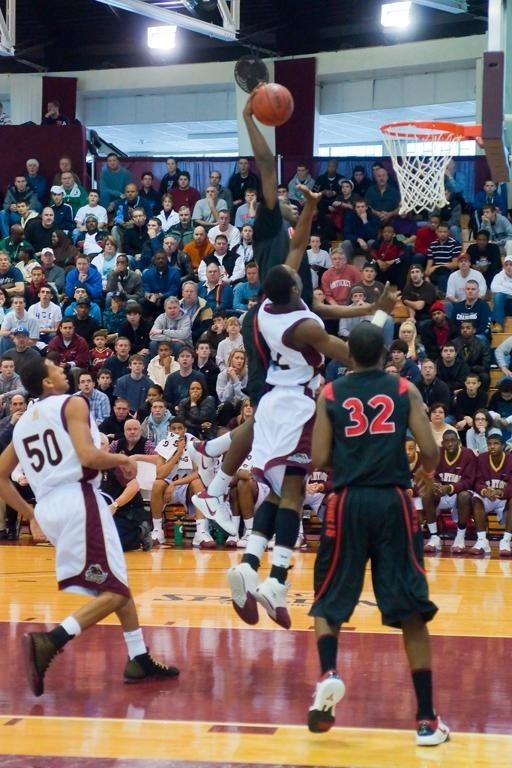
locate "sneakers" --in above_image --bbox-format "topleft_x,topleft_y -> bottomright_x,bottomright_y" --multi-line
148,529 -> 166,546
188,440 -> 219,489
124,652 -> 179,682
225,562 -> 258,624
191,487 -> 253,547
423,535 -> 512,556
308,668 -> 345,731
252,576 -> 291,628
415,715 -> 450,745
492,322 -> 503,333
24,632 -> 58,696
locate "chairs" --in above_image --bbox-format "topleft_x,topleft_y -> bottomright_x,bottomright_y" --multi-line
5,489 -> 512,546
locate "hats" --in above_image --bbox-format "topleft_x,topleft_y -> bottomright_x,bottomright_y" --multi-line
76,299 -> 90,306
504,255 -> 512,263
431,303 -> 446,313
13,327 -> 28,335
42,248 -> 53,256
458,253 -> 469,261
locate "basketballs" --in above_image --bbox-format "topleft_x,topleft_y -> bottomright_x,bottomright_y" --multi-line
250,83 -> 293,125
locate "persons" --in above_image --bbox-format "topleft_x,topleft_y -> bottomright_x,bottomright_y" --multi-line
0,357 -> 180,697
226,184 -> 351,628
308,321 -> 451,746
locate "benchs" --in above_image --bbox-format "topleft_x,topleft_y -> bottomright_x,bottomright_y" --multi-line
376,271 -> 512,413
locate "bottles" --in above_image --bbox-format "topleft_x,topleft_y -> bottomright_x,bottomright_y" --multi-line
174,519 -> 184,546
215,528 -> 225,545
